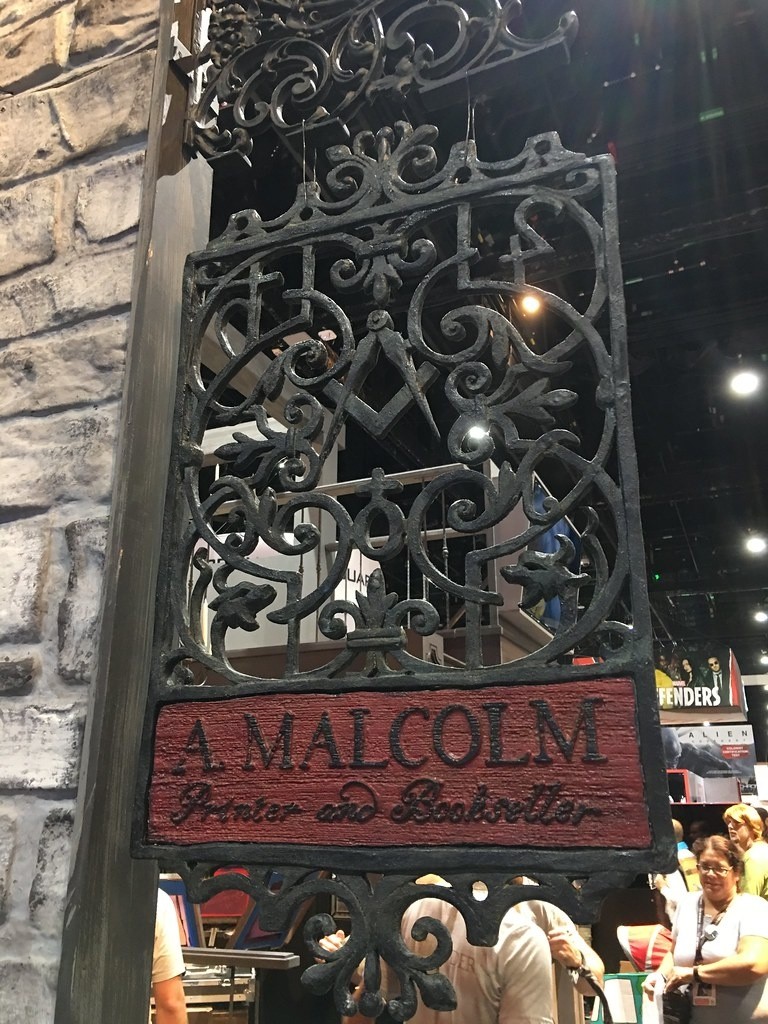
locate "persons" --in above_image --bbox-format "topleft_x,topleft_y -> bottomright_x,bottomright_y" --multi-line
146,793 -> 768,1023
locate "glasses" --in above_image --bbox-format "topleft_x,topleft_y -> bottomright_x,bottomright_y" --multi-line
695,864 -> 734,877
